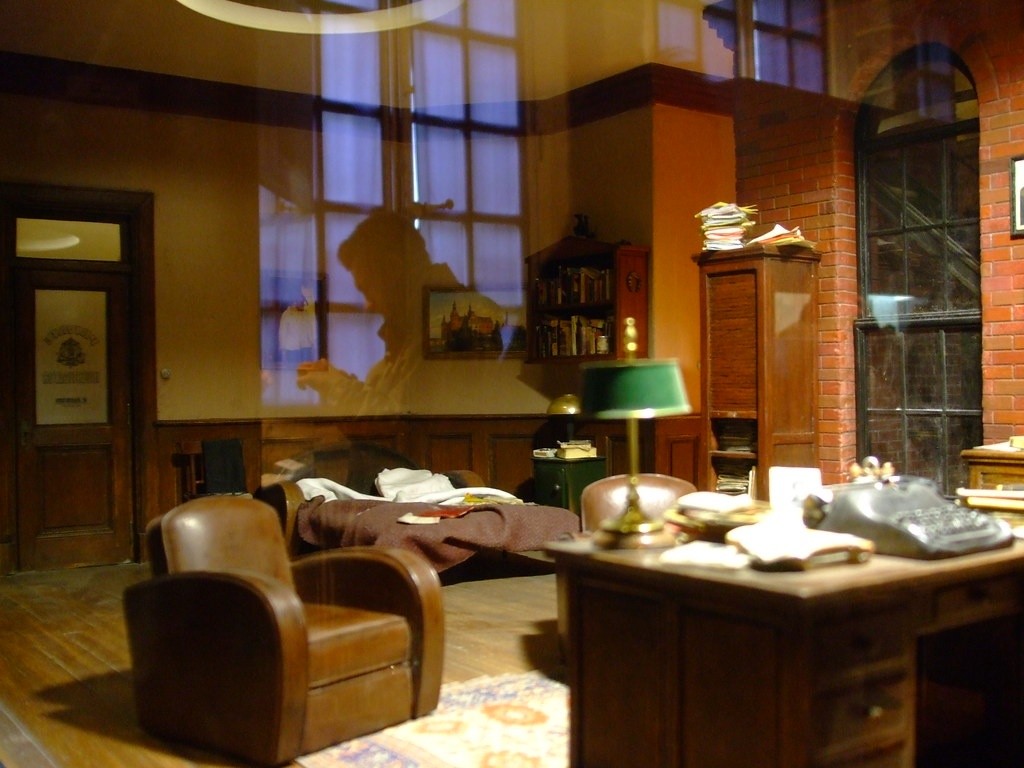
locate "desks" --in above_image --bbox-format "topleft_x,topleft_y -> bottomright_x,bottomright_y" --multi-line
960,436 -> 1024,492
543,506 -> 1024,768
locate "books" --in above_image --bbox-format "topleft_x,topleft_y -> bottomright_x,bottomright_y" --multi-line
694,201 -> 816,250
533,265 -> 615,357
715,422 -> 759,500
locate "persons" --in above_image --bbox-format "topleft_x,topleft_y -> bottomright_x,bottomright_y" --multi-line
297,211 -> 505,412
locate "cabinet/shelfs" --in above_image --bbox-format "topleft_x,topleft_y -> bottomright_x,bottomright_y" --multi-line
686,244 -> 824,500
520,234 -> 649,367
531,455 -> 607,519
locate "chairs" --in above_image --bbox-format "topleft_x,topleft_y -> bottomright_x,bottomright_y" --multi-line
580,473 -> 697,533
176,436 -> 249,505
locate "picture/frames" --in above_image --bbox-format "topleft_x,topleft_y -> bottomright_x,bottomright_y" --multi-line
423,283 -> 528,360
259,266 -> 329,371
1008,155 -> 1024,239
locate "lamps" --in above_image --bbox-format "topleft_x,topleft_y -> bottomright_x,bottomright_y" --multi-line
545,393 -> 585,443
579,318 -> 694,558
277,217 -> 322,353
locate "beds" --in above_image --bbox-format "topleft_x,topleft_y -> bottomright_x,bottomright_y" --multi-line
255,438 -> 579,574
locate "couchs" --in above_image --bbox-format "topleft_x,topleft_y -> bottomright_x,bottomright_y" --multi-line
119,494 -> 444,764
